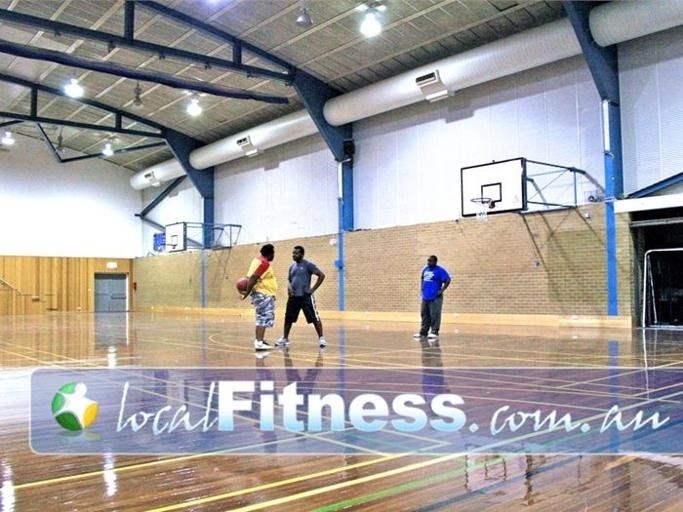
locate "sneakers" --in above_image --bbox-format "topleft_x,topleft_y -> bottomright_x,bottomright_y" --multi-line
319,336 -> 327,347
413,333 -> 438,338
254,337 -> 290,351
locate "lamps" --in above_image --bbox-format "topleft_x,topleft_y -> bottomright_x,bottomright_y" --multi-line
55,135 -> 65,152
132,83 -> 143,107
295,8 -> 314,27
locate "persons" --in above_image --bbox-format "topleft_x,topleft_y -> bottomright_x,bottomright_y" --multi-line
249,350 -> 279,456
280,346 -> 326,443
412,336 -> 451,431
412,255 -> 451,338
237,244 -> 276,350
273,245 -> 328,348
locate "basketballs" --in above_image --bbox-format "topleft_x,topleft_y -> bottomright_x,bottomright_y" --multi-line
236,278 -> 250,291
